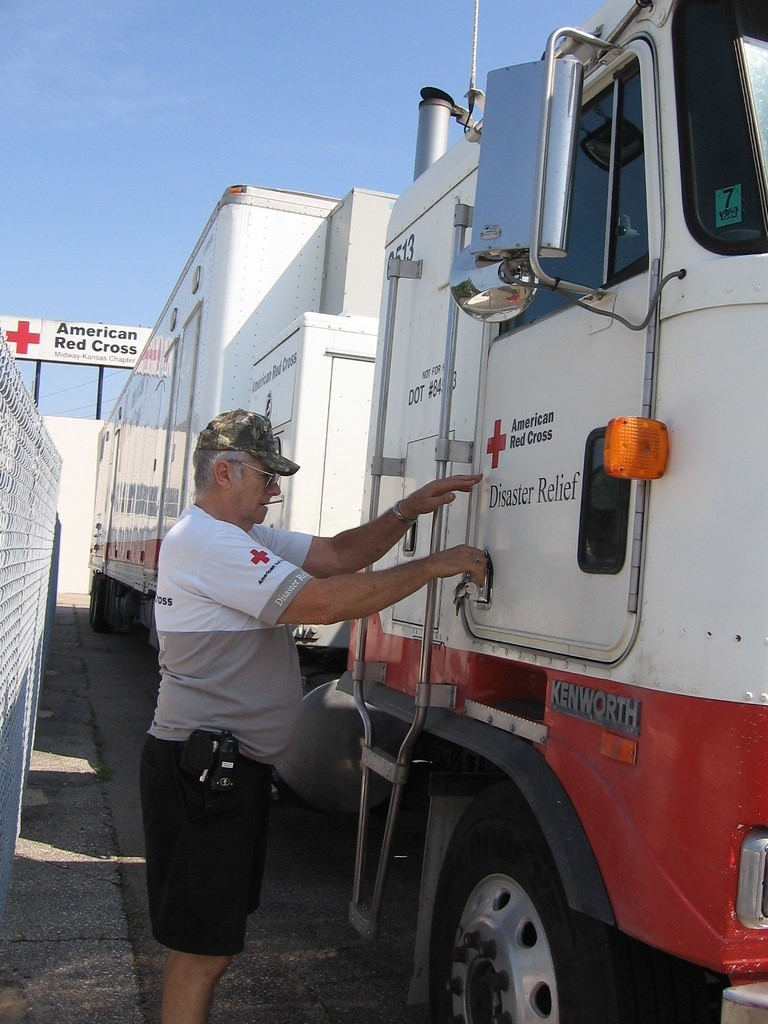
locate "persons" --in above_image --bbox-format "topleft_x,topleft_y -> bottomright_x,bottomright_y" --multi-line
140,408 -> 490,1024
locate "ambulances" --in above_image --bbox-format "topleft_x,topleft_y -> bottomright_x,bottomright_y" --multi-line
84,1 -> 768,1023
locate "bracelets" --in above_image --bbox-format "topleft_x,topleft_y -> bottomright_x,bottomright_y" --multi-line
393,498 -> 418,525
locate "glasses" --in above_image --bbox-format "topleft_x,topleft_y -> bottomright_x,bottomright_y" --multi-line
228,459 -> 280,488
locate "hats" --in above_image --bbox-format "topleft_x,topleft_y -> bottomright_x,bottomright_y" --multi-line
196,408 -> 300,476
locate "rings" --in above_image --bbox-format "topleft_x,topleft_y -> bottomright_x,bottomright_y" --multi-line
475,559 -> 480,565
